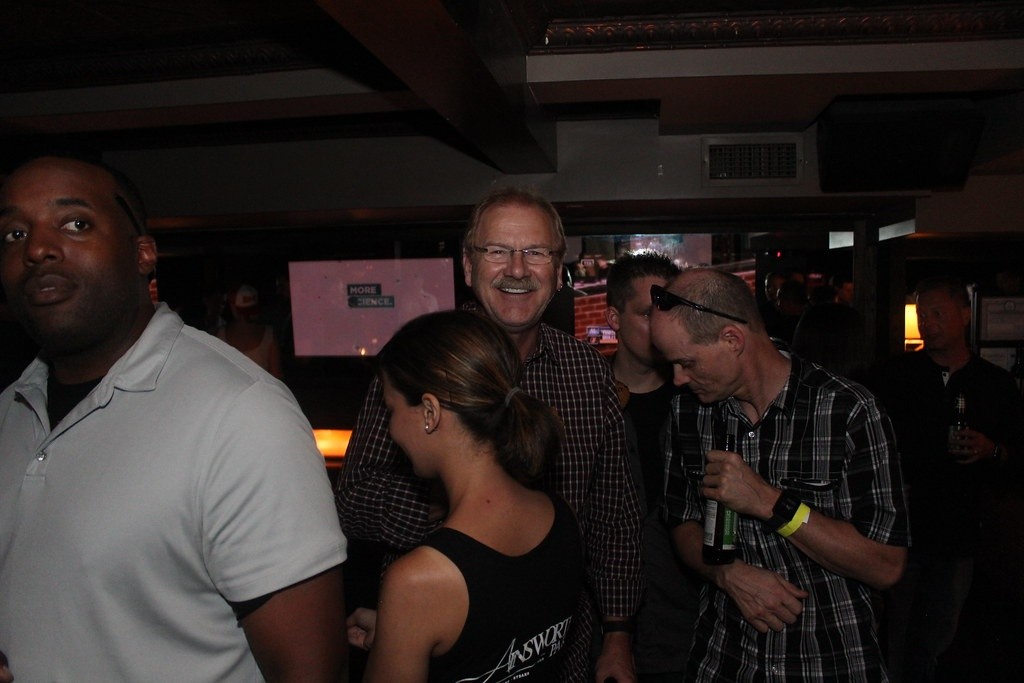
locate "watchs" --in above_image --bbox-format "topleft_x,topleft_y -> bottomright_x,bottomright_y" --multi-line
759,491 -> 800,536
988,441 -> 1002,464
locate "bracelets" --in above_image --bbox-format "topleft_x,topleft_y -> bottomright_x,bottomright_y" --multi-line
601,622 -> 636,636
776,502 -> 809,536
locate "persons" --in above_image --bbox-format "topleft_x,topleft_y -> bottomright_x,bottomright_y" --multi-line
216,282 -> 284,378
0,154 -> 348,683
347,311 -> 582,683
650,270 -> 912,682
762,271 -> 1024,683
605,254 -> 701,683
338,187 -> 646,683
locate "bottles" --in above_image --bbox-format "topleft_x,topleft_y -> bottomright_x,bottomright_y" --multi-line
702,434 -> 738,566
949,394 -> 968,461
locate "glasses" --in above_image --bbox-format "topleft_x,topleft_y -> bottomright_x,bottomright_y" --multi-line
474,245 -> 562,265
649,284 -> 748,324
